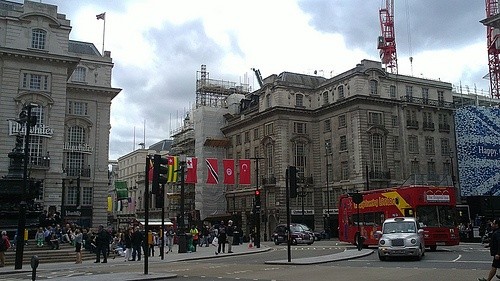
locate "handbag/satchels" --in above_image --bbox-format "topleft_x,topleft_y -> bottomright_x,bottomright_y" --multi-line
193,234 -> 198,240
212,237 -> 218,246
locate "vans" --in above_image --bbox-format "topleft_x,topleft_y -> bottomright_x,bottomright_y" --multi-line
375,217 -> 425,261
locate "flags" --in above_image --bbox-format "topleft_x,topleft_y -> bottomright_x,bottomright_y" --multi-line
205,158 -> 219,184
185,157 -> 198,183
223,159 -> 235,185
96,13 -> 104,20
239,159 -> 250,185
166,156 -> 179,182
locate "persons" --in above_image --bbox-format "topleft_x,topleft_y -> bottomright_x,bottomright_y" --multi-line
458,220 -> 495,249
477,219 -> 500,281
148,220 -> 254,257
0,229 -> 18,267
24,210 -> 146,264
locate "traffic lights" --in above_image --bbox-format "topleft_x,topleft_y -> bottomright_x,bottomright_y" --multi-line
151,153 -> 169,194
254,190 -> 260,205
289,165 -> 301,198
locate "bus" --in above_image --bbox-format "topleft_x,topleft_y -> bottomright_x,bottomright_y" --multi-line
338,184 -> 463,251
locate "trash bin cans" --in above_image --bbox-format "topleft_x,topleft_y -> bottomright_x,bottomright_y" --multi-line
179,233 -> 196,253
232,232 -> 239,245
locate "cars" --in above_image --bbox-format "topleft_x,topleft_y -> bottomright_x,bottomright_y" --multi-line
271,223 -> 315,246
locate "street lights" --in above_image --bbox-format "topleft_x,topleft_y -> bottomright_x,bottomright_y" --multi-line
13,102 -> 39,270
178,150 -> 187,253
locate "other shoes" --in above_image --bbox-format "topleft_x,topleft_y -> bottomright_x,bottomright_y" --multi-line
101,261 -> 107,263
228,251 -> 233,253
41,244 -> 43,247
37,245 -> 40,247
94,261 -> 100,263
130,259 -> 135,261
75,260 -> 82,264
137,259 -> 141,261
